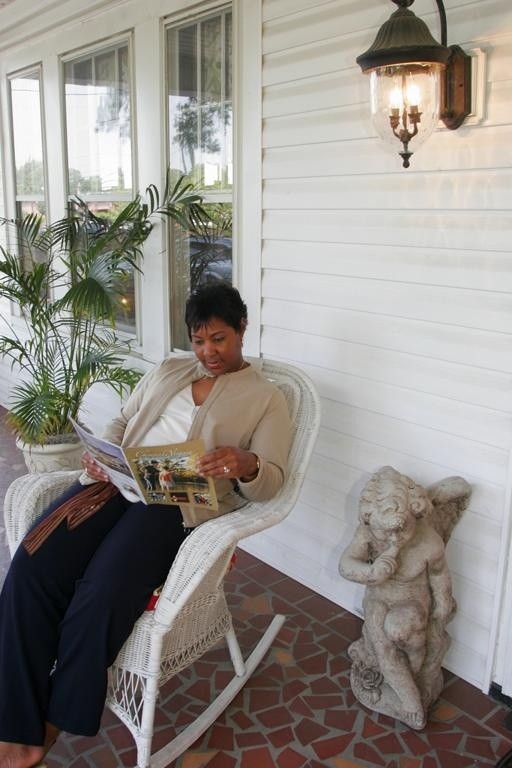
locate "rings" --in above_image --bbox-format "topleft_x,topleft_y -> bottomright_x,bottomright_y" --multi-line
223,465 -> 230,473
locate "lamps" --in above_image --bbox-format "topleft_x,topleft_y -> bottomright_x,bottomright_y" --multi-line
357,0 -> 473,168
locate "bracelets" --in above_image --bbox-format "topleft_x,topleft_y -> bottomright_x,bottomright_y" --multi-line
243,453 -> 260,480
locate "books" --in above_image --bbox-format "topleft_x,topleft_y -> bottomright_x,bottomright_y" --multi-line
69,415 -> 219,513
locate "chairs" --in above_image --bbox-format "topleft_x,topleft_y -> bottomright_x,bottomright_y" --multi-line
2,357 -> 324,767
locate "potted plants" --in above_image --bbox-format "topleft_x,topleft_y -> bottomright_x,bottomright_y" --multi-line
0,162 -> 216,477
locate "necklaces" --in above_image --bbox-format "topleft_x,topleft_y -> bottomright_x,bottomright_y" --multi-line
238,360 -> 246,370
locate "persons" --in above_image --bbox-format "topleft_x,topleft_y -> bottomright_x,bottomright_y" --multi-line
138,460 -> 160,491
338,465 -> 472,728
0,285 -> 295,767
159,465 -> 177,491
